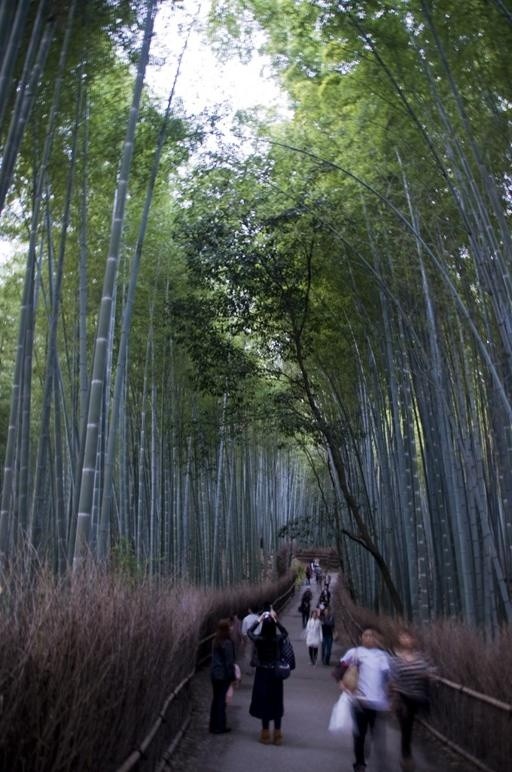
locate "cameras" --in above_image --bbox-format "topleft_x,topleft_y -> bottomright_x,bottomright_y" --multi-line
263,611 -> 270,619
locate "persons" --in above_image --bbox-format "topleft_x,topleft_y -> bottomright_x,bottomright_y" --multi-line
305,555 -> 331,588
209,618 -> 237,734
243,604 -> 264,674
320,606 -> 336,666
319,583 -> 331,607
231,611 -> 242,661
390,627 -> 431,771
247,608 -> 289,744
300,585 -> 312,628
305,610 -> 323,669
337,624 -> 396,771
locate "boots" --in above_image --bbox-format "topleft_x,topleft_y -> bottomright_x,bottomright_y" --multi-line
257,729 -> 272,746
272,729 -> 284,746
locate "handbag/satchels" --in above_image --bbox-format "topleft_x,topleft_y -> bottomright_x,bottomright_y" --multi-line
342,662 -> 360,692
270,660 -> 291,681
327,690 -> 356,737
282,635 -> 296,670
